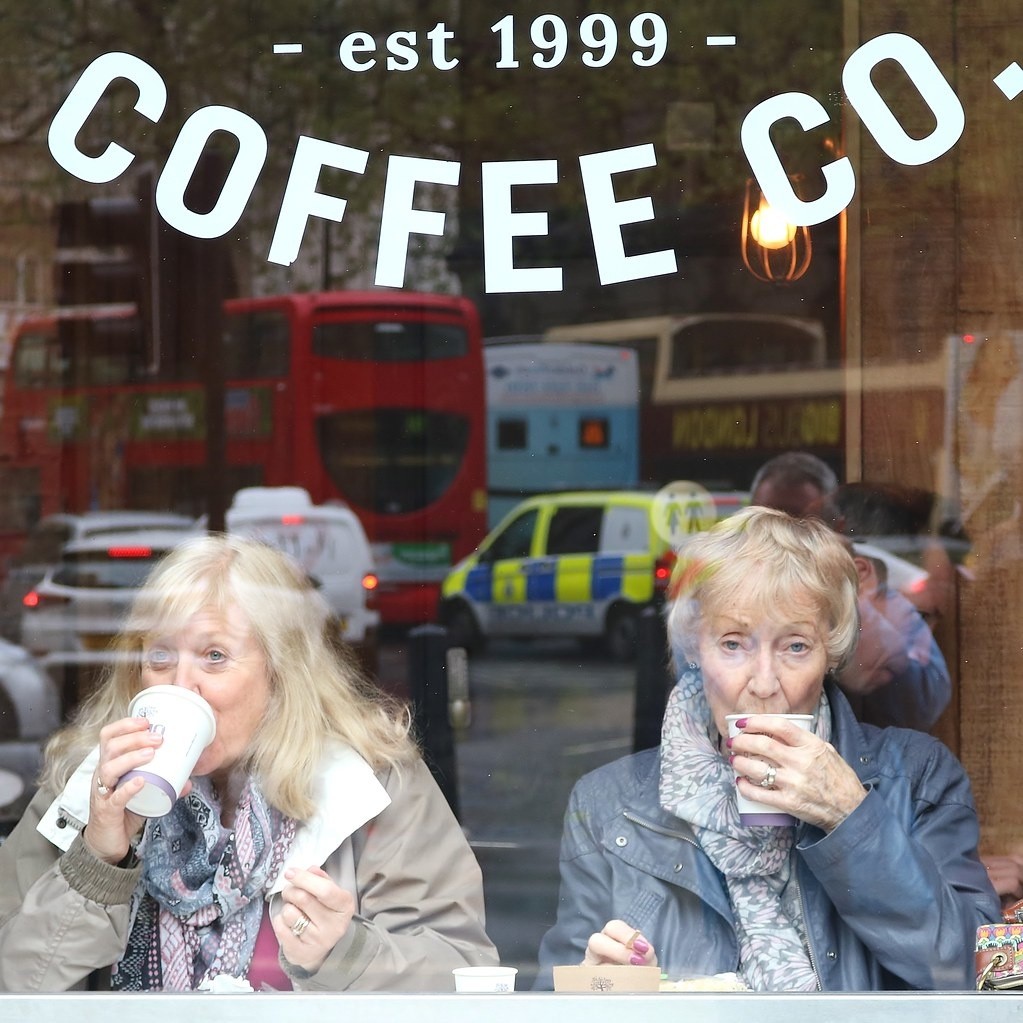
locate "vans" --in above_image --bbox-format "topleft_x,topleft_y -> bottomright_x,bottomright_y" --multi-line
437,491 -> 753,661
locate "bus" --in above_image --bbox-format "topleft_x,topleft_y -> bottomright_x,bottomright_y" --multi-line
0,291 -> 485,640
484,344 -> 642,537
546,313 -> 831,492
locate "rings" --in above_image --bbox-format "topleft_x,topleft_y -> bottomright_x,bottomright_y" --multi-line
761,765 -> 776,786
292,914 -> 311,935
97,777 -> 107,795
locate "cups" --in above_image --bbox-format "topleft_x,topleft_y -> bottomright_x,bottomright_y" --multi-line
724,714 -> 815,827
451,967 -> 517,993
114,685 -> 217,817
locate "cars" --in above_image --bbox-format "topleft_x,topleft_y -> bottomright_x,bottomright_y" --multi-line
0,510 -> 207,668
225,485 -> 379,674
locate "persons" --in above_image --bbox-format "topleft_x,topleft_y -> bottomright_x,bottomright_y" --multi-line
0,537 -> 500,995
750,454 -> 974,729
529,505 -> 1003,991
